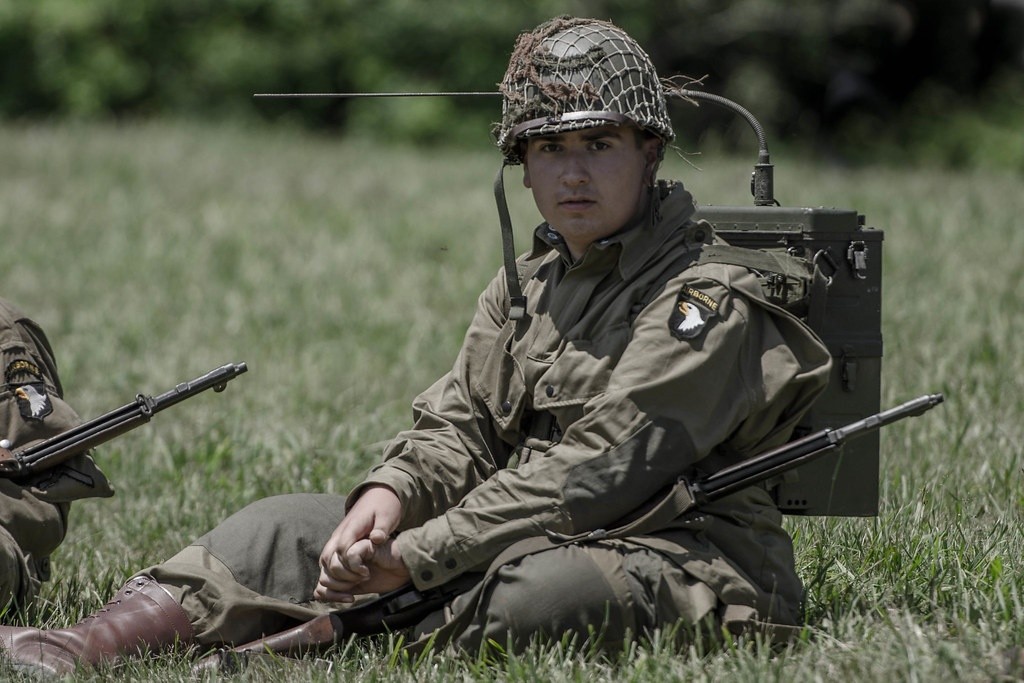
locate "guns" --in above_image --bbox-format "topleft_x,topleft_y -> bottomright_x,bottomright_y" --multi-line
0,362 -> 251,490
187,392 -> 948,676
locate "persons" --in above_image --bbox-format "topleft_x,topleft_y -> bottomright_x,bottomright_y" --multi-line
1,14 -> 833,681
0,299 -> 113,629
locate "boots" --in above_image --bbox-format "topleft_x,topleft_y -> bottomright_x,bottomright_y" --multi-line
0,577 -> 191,681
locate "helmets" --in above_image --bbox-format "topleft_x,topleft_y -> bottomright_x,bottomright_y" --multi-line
495,19 -> 674,161
